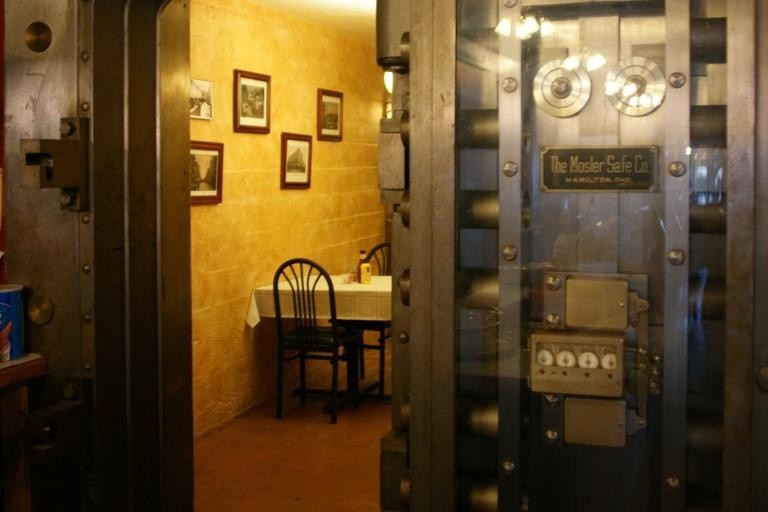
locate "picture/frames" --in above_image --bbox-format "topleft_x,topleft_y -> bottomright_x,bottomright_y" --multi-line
317,88 -> 343,142
189,79 -> 214,119
281,133 -> 312,189
191,142 -> 223,205
234,69 -> 270,135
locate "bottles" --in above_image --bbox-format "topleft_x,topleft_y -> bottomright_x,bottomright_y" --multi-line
352,249 -> 372,285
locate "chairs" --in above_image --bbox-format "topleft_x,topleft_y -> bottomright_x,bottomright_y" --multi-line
361,243 -> 391,381
273,258 -> 356,424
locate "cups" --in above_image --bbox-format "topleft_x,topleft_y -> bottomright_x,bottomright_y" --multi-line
0,284 -> 24,363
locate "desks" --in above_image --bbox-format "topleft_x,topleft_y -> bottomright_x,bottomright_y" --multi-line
245,273 -> 392,414
1,353 -> 48,512
